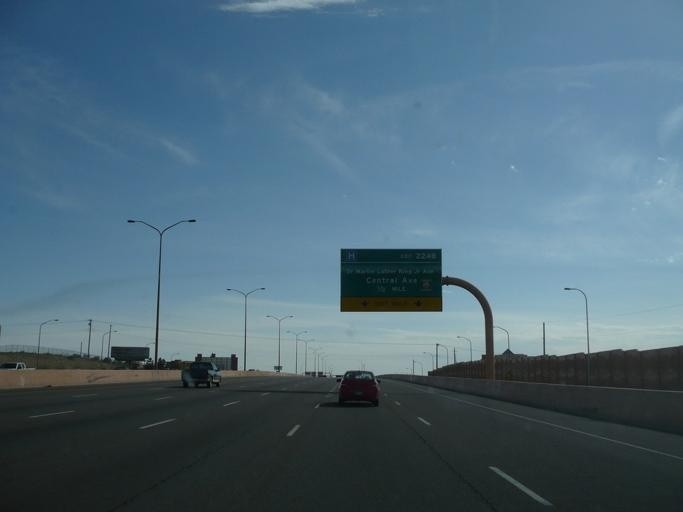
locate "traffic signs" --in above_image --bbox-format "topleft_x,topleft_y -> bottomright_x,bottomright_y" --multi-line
339,247 -> 443,313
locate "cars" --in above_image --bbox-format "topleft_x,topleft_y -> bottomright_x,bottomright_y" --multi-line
335,369 -> 383,408
248,368 -> 256,371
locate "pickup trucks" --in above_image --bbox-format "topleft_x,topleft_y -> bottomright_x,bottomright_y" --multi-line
0,361 -> 36,370
178,360 -> 222,390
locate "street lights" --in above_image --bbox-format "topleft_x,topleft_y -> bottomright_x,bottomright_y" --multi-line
226,287 -> 266,371
170,352 -> 180,361
563,287 -> 589,355
36,317 -> 120,364
266,314 -> 295,372
283,328 -> 335,378
144,342 -> 156,365
492,326 -> 510,350
455,336 -> 473,362
123,216 -> 198,369
404,342 -> 449,377
